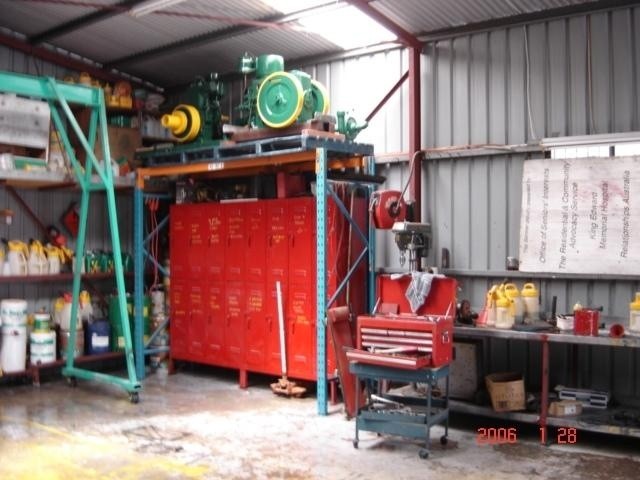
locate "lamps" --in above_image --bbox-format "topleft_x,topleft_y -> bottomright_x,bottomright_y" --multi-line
127,1 -> 184,18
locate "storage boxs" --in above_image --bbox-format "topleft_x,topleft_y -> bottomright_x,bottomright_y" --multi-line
485,371 -> 525,413
76,124 -> 142,175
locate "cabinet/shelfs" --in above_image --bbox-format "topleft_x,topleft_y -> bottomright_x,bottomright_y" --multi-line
379,321 -> 639,444
133,134 -> 384,415
352,362 -> 450,459
169,197 -> 367,404
2,167 -> 170,387
